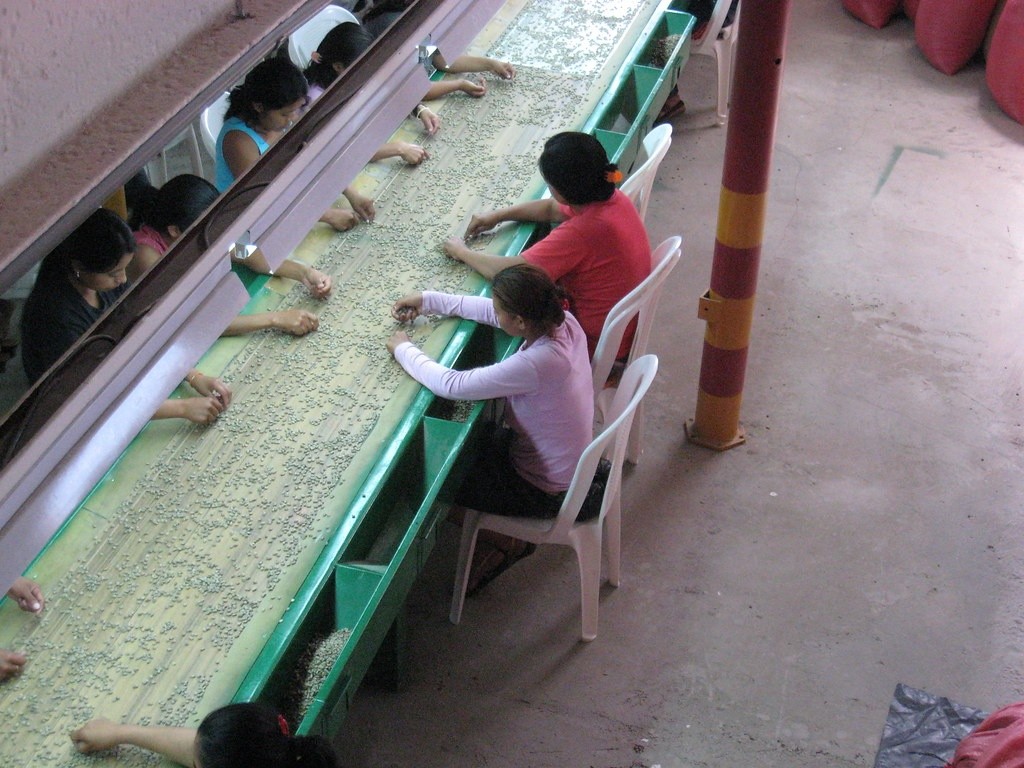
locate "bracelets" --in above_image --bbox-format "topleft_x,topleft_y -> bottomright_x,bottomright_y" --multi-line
413,103 -> 430,120
189,372 -> 203,388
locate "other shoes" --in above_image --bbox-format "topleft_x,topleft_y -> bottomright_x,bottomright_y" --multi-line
448,540 -> 537,598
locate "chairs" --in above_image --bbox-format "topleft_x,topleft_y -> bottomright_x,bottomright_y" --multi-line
199,92 -> 231,163
449,354 -> 658,642
144,124 -> 205,189
288,4 -> 360,70
591,236 -> 683,464
691,0 -> 742,126
618,123 -> 673,221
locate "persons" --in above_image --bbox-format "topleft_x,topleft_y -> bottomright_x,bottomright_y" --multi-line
301,21 -> 440,165
213,57 -> 375,232
70,702 -> 337,768
20,208 -> 232,427
0,576 -> 45,682
362,0 -> 517,101
443,131 -> 652,376
386,263 -> 595,521
127,174 -> 332,336
